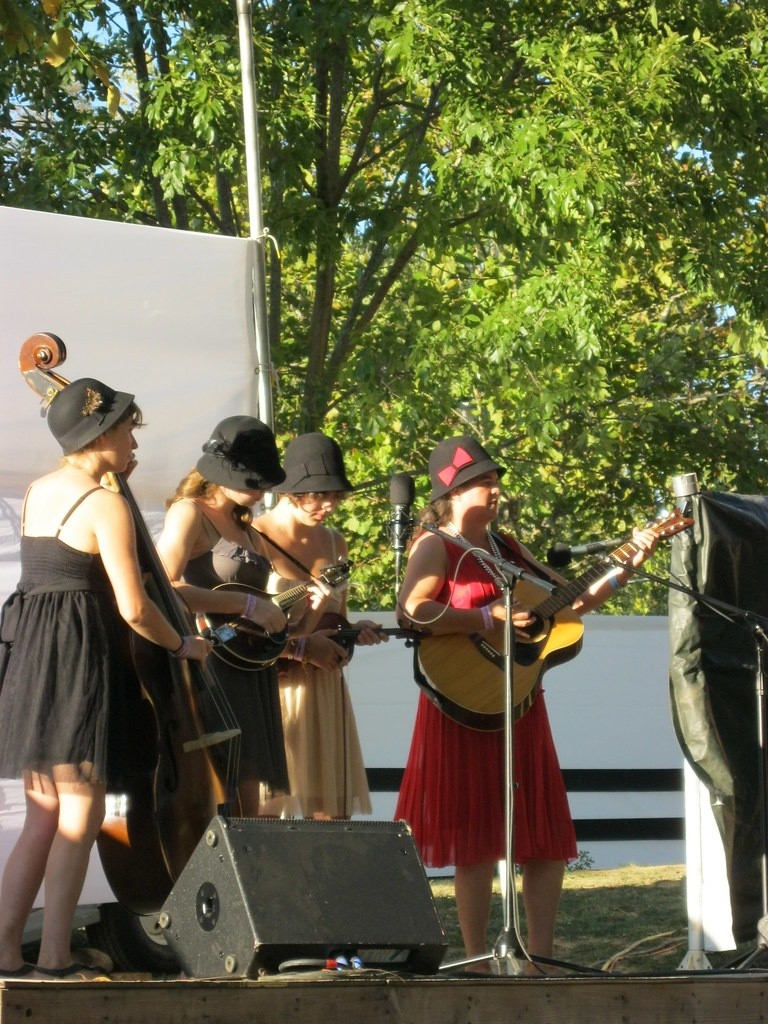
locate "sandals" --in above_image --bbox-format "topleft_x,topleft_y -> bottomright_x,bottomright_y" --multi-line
34,962 -> 110,979
0,962 -> 34,979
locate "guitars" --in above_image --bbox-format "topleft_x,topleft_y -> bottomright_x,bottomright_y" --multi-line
193,555 -> 359,673
411,511 -> 695,734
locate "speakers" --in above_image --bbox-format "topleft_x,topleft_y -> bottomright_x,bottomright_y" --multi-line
155,816 -> 451,979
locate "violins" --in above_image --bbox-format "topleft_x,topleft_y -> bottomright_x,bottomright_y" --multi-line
264,611 -> 429,674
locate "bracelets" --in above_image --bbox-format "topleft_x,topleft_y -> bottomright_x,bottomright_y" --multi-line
478,604 -> 496,634
287,639 -> 296,660
606,569 -> 624,591
242,594 -> 256,619
169,636 -> 191,659
294,637 -> 305,661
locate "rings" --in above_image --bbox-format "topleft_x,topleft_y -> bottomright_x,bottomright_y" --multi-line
205,653 -> 208,656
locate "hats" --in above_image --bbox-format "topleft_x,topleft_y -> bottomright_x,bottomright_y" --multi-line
47,377 -> 135,456
270,433 -> 354,493
196,415 -> 287,490
428,436 -> 507,503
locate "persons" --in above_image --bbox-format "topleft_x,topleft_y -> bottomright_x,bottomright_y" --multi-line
393,436 -> 659,976
153,415 -> 332,819
0,378 -> 212,983
253,432 -> 388,970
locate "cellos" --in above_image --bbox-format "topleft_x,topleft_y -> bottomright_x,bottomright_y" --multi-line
17,330 -> 262,918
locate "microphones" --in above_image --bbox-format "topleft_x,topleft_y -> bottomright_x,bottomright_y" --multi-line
390,473 -> 415,575
545,537 -> 624,567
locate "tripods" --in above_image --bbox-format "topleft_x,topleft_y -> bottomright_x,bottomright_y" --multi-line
590,551 -> 768,974
408,516 -> 608,974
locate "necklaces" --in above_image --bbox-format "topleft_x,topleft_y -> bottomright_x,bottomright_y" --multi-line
445,522 -> 505,576
